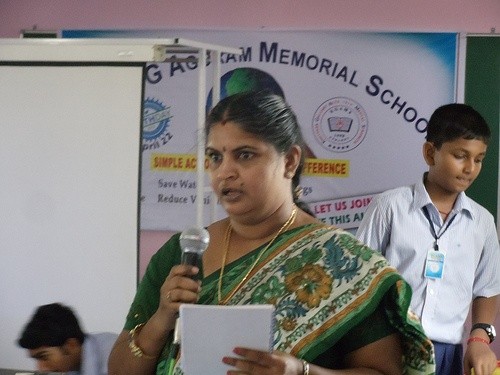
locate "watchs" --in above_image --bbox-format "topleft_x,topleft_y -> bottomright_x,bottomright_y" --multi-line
470,323 -> 496,344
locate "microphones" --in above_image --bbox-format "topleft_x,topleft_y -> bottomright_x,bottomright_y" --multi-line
171,225 -> 211,360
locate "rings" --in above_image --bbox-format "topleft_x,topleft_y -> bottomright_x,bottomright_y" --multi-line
167,290 -> 171,301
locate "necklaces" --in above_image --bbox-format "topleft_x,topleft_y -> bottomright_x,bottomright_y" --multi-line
439,210 -> 449,216
217,203 -> 297,305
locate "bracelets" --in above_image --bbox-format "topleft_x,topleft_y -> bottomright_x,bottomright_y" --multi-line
127,323 -> 159,360
467,337 -> 489,345
301,359 -> 310,375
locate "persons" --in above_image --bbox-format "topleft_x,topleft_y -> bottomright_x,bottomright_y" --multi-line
357,103 -> 500,375
108,89 -> 437,375
17,303 -> 120,375
